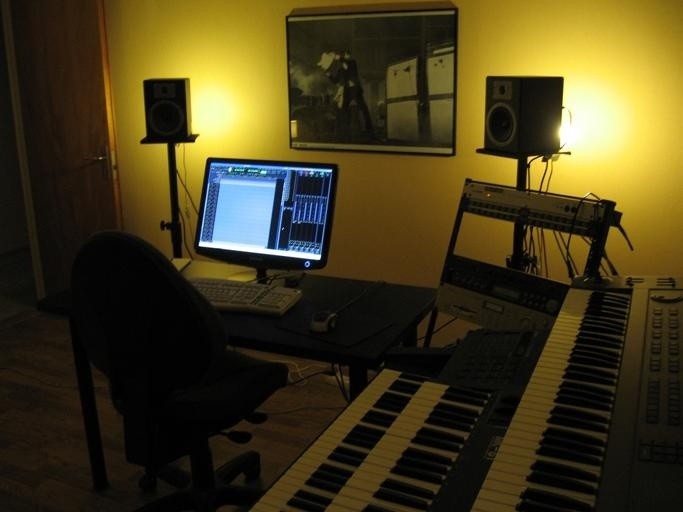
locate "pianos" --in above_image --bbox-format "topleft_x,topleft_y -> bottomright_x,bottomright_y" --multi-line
249,285 -> 682,511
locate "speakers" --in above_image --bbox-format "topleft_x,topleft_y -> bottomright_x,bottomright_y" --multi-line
144,78 -> 192,139
485,76 -> 564,156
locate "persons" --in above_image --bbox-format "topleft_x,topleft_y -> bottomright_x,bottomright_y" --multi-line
324,48 -> 376,134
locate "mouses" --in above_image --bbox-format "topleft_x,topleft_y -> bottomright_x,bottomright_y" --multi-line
310,310 -> 338,333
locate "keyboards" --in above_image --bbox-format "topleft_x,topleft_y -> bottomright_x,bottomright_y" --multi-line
185,278 -> 303,316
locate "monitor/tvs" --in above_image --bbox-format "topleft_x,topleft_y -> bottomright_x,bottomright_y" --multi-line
193,157 -> 338,285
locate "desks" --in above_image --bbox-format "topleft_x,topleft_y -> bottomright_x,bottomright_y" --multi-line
66,255 -> 442,494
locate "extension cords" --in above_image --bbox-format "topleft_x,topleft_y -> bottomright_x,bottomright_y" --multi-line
325,374 -> 349,390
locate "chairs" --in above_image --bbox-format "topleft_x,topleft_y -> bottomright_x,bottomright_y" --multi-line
66,226 -> 288,512
